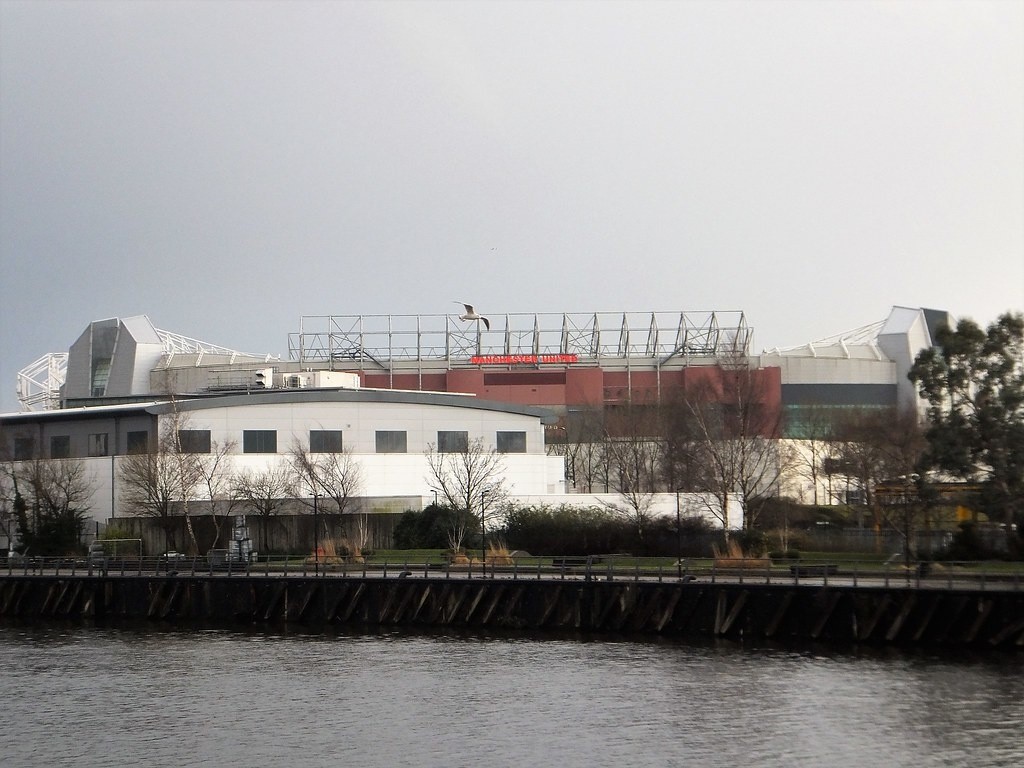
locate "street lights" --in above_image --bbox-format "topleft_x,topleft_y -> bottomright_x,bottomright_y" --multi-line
164,498 -> 173,575
430,489 -> 437,507
676,487 -> 684,581
309,492 -> 322,576
482,490 -> 490,575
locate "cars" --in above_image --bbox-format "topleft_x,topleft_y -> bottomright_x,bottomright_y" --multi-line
164,551 -> 180,558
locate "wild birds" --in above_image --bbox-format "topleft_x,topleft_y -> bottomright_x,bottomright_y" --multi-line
453,301 -> 490,332
